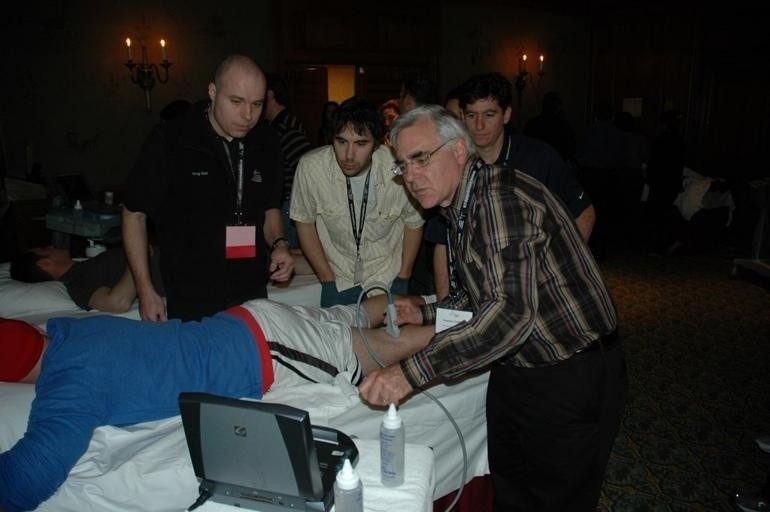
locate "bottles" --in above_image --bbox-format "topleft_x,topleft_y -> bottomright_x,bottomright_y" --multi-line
333,456 -> 364,512
72,200 -> 84,224
380,403 -> 407,488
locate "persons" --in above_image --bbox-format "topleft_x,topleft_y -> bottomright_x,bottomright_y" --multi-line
260,70 -> 315,251
1,285 -> 446,511
118,51 -> 296,323
9,242 -> 171,312
290,71 -> 656,321
353,103 -> 621,509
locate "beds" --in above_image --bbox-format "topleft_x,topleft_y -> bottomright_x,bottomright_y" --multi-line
12,256 -> 325,321
1,307 -> 494,511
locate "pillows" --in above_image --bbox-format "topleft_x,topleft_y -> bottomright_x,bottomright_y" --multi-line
0,261 -> 81,316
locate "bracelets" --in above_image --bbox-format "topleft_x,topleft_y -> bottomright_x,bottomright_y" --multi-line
271,235 -> 287,248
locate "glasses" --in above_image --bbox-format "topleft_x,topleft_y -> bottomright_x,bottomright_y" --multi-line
390,142 -> 449,175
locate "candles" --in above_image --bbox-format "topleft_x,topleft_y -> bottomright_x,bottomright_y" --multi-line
539,55 -> 544,71
522,54 -> 527,72
126,37 -> 132,60
160,38 -> 166,60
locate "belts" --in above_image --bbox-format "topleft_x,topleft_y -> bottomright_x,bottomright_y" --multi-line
574,343 -> 599,356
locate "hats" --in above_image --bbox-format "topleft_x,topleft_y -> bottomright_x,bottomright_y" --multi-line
1,318 -> 44,383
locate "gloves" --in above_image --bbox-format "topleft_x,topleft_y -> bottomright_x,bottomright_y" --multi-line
320,281 -> 344,309
390,275 -> 409,296
